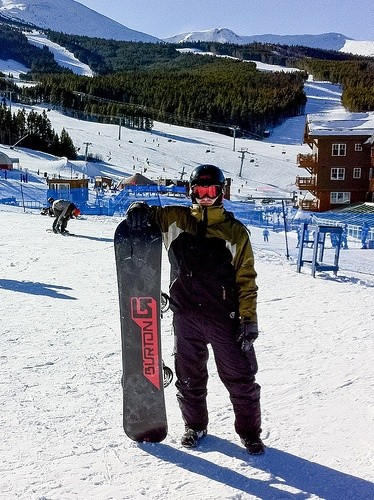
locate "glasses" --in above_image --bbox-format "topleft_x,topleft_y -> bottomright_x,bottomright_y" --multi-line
192,185 -> 222,199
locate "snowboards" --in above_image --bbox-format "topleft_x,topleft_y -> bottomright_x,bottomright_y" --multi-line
109,213 -> 170,444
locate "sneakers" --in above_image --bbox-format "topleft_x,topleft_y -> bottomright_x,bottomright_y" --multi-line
241,434 -> 265,455
181,425 -> 208,448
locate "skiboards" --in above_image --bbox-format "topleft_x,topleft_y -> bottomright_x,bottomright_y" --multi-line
46,228 -> 73,237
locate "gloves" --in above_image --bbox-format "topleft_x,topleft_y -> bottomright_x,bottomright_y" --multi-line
125,199 -> 152,232
236,322 -> 259,351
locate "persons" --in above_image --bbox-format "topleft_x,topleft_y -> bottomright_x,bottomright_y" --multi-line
53,200 -> 79,236
263,227 -> 269,242
296,223 -> 348,249
126,165 -> 265,455
358,222 -> 370,249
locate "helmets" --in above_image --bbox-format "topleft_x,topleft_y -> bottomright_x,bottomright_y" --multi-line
75,209 -> 80,215
185,163 -> 229,192
